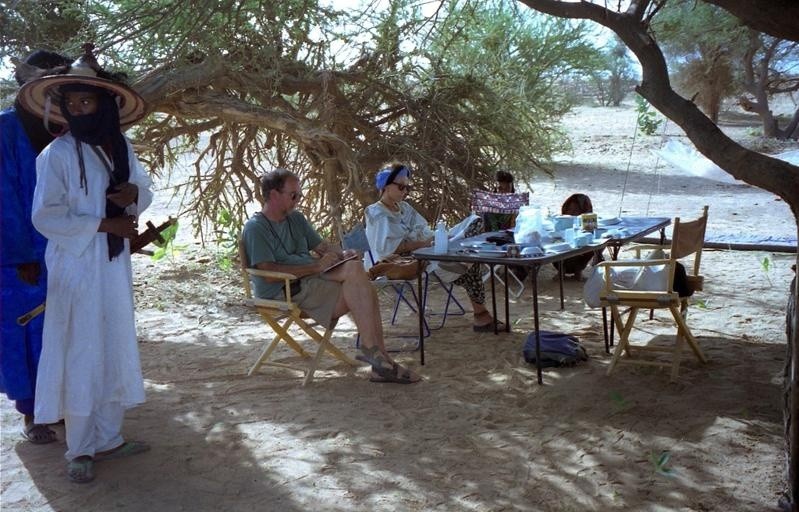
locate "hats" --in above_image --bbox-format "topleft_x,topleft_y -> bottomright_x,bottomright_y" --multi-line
17,42 -> 147,129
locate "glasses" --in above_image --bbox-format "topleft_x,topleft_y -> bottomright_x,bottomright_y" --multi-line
277,188 -> 303,201
392,181 -> 412,191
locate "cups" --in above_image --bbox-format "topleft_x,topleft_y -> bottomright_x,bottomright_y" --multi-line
564,228 -> 575,242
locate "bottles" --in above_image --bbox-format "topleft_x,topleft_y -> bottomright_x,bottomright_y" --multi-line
434,219 -> 448,255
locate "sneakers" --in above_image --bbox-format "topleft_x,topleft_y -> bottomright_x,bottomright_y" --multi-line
438,261 -> 467,274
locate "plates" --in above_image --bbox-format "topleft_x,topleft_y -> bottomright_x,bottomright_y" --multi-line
477,250 -> 508,257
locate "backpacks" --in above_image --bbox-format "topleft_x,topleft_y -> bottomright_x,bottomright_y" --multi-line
523,327 -> 588,370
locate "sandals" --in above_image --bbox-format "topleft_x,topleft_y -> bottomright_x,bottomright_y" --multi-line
355,344 -> 396,372
369,365 -> 421,384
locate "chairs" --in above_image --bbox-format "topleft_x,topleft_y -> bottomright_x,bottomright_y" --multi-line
344,223 -> 463,337
235,230 -> 356,388
469,186 -> 530,300
583,204 -> 713,383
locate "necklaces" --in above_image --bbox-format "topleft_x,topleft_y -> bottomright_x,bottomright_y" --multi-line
259,209 -> 302,258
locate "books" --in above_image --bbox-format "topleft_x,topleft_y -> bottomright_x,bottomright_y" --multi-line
323,255 -> 357,273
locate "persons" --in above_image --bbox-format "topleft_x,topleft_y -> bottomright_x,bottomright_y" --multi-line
360,162 -> 509,333
477,170 -> 531,288
552,193 -> 603,281
238,168 -> 423,386
0,102 -> 70,448
31,82 -> 156,486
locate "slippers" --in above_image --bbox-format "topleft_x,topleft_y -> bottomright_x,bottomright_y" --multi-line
19,420 -> 57,444
102,440 -> 152,460
473,319 -> 510,332
65,460 -> 95,483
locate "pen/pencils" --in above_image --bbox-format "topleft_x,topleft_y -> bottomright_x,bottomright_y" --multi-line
313,249 -> 324,257
